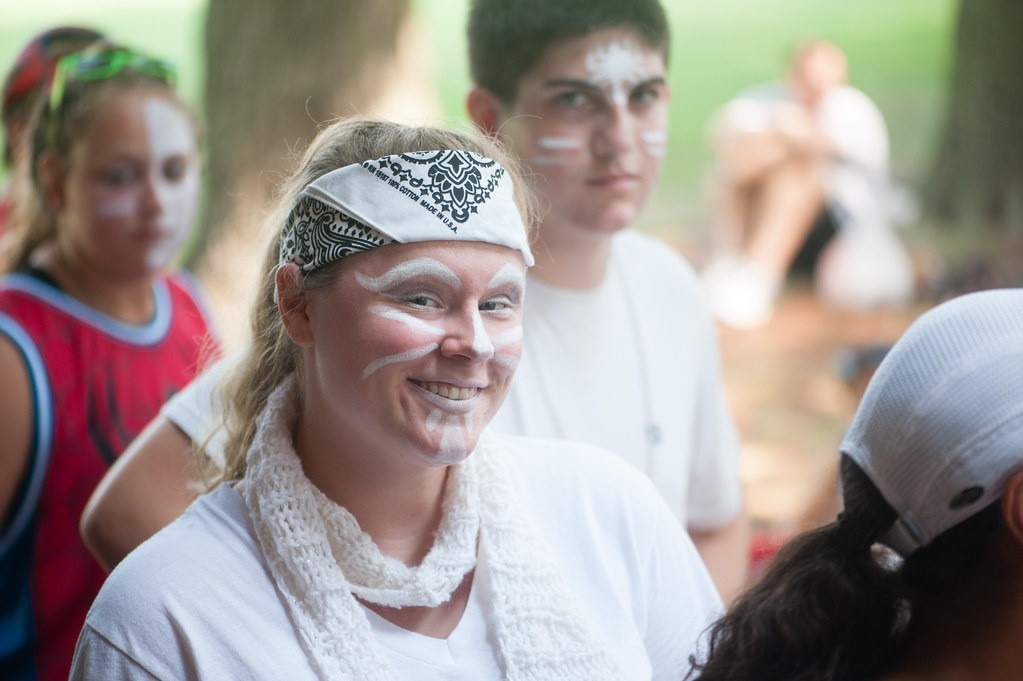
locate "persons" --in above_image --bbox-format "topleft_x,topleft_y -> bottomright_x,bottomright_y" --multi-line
66,121 -> 728,681
705,42 -> 890,325
89,0 -> 749,610
695,290 -> 1023,681
0,27 -> 223,681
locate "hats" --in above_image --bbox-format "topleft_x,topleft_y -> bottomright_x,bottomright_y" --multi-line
838,287 -> 1022,571
